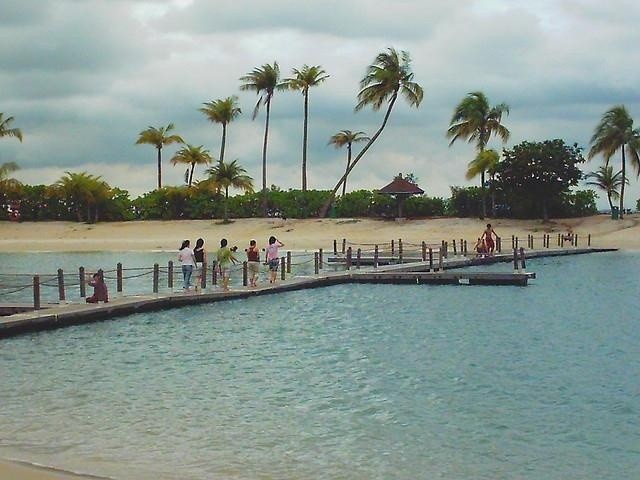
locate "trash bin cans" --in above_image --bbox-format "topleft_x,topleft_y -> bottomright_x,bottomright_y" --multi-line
330,204 -> 338,218
612,206 -> 619,219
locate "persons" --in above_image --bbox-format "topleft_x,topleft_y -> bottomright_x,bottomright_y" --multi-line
265,236 -> 285,283
177,240 -> 198,288
480,224 -> 498,255
193,238 -> 208,284
474,237 -> 487,253
86,273 -> 108,303
246,240 -> 260,287
216,238 -> 234,291
